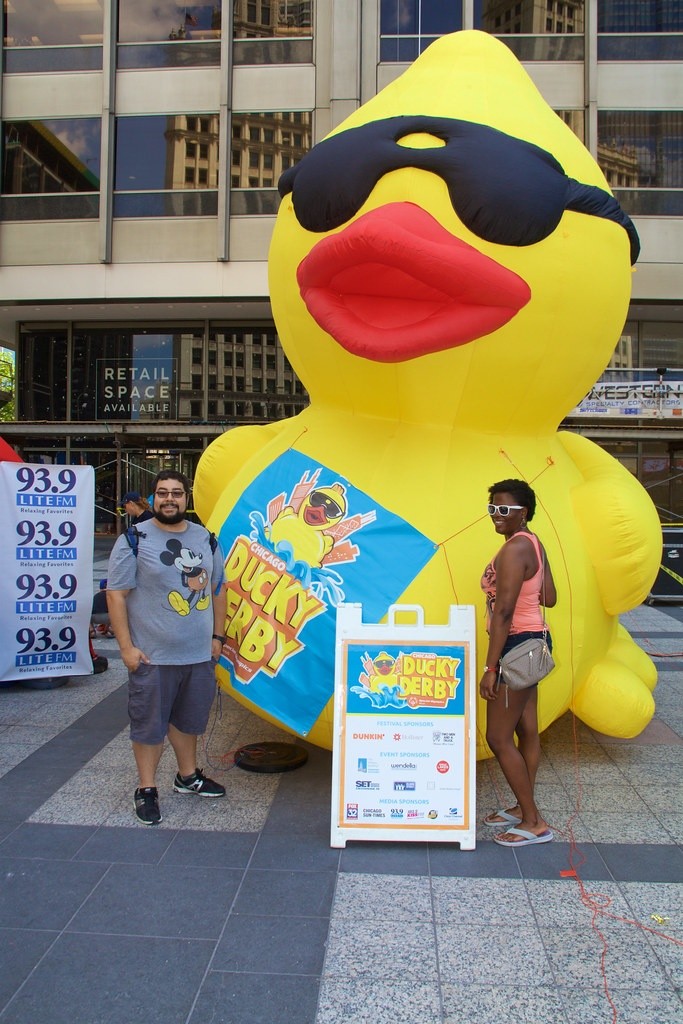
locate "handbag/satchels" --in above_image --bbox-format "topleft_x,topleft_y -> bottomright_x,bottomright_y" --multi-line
498,639 -> 555,691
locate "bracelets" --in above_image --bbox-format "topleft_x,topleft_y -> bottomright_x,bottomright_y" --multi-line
213,634 -> 225,645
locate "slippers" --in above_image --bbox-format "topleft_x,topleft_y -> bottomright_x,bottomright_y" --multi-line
483,808 -> 523,826
494,826 -> 554,846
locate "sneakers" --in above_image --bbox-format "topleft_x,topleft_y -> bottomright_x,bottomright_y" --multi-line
133,785 -> 163,825
171,767 -> 225,797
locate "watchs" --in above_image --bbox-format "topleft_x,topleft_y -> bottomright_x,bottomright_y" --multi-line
484,666 -> 498,673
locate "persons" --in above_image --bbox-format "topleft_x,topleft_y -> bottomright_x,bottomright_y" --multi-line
106,471 -> 227,825
115,493 -> 155,522
479,479 -> 558,848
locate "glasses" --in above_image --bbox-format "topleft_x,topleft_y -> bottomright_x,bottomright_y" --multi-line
153,489 -> 186,498
487,503 -> 522,517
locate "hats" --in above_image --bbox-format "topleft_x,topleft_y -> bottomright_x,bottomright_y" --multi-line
115,491 -> 141,505
